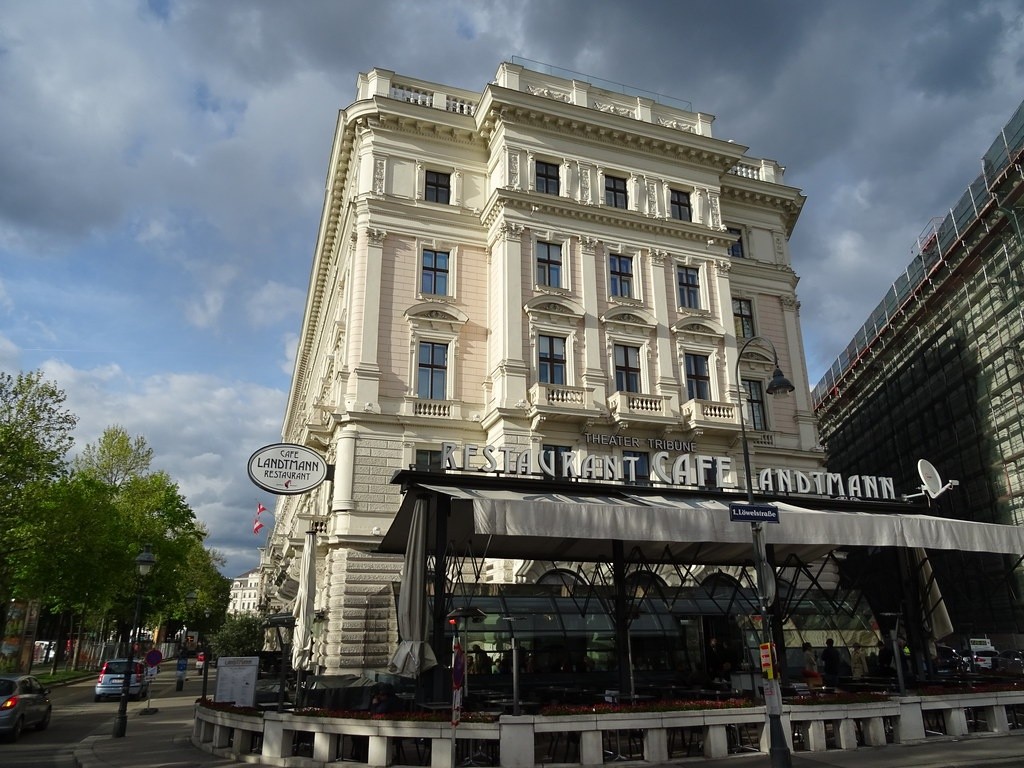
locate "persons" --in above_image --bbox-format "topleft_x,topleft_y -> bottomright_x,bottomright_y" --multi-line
877,640 -> 892,666
196,652 -> 204,676
802,641 -> 818,672
891,639 -> 911,672
819,638 -> 840,674
465,645 -> 527,674
851,642 -> 863,677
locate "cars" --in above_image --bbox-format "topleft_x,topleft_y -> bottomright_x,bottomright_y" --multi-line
973,650 -> 1000,672
990,649 -> 1024,677
0,673 -> 52,743
938,645 -> 972,673
937,660 -> 963,680
94,659 -> 148,701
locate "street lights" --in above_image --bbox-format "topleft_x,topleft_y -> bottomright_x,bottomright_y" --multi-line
202,607 -> 212,650
735,334 -> 797,768
175,589 -> 199,690
111,550 -> 157,736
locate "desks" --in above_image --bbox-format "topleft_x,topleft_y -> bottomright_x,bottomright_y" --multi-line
252,673 -> 1024,767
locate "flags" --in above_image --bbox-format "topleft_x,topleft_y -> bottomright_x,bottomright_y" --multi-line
253,518 -> 264,535
257,504 -> 265,515
